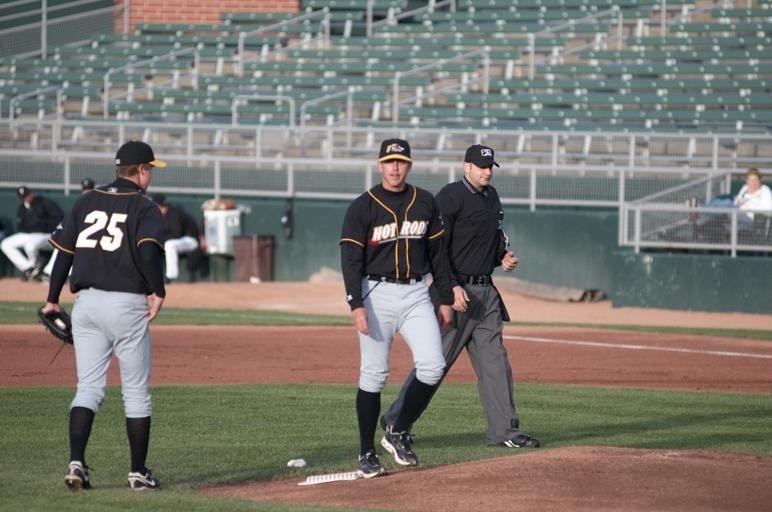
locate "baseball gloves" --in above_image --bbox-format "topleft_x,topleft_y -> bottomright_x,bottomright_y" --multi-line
39,303 -> 74,345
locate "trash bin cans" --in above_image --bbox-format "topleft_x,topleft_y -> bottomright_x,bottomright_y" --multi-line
233,235 -> 273,281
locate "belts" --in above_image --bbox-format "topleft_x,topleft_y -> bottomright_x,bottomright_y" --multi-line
368,274 -> 422,284
457,274 -> 490,287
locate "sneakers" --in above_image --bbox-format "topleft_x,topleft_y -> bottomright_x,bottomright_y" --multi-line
380,426 -> 418,467
64,461 -> 94,490
502,435 -> 540,448
380,415 -> 416,444
127,469 -> 159,491
357,450 -> 386,479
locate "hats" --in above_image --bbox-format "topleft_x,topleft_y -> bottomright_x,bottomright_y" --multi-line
378,138 -> 413,163
82,178 -> 94,190
16,186 -> 28,199
114,141 -> 166,168
464,144 -> 500,167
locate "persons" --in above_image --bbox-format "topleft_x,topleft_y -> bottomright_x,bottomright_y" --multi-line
145,193 -> 200,286
39,137 -> 168,492
338,136 -> 454,480
683,166 -> 770,234
0,185 -> 65,284
40,178 -> 96,282
378,142 -> 540,449
